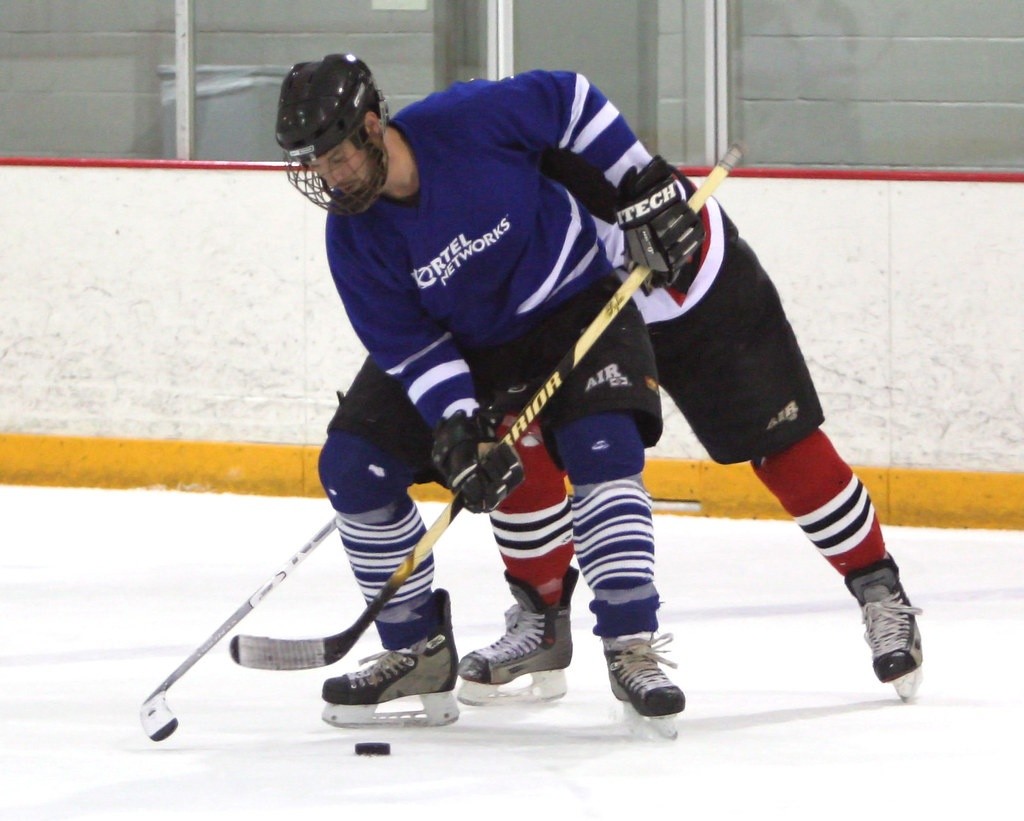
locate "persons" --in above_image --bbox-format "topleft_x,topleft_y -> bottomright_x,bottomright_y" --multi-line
274,50 -> 709,717
457,166 -> 924,687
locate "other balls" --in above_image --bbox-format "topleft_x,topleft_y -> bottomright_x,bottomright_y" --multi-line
354,741 -> 391,756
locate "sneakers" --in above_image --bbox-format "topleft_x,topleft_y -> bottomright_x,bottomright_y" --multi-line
844,550 -> 921,704
455,566 -> 579,705
601,627 -> 687,741
321,589 -> 460,730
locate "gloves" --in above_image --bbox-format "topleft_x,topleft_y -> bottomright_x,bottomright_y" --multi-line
427,406 -> 526,515
612,154 -> 707,296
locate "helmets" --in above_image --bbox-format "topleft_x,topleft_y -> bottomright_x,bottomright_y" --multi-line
274,54 -> 387,216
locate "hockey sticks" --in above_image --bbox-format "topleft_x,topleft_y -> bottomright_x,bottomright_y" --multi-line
225,139 -> 747,673
138,516 -> 340,744
